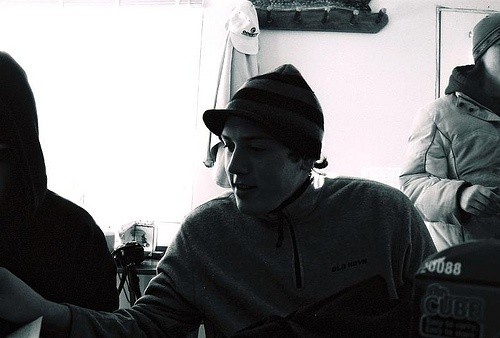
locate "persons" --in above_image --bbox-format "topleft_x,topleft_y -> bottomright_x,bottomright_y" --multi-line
0,64 -> 437,338
398,12 -> 500,253
0,51 -> 120,338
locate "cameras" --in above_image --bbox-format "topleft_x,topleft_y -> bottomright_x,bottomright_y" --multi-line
111,241 -> 144,268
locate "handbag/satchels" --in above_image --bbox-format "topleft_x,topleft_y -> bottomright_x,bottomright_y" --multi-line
112,241 -> 146,268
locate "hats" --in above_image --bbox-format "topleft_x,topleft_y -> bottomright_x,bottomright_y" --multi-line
229,1 -> 260,55
203,63 -> 324,160
472,13 -> 500,63
